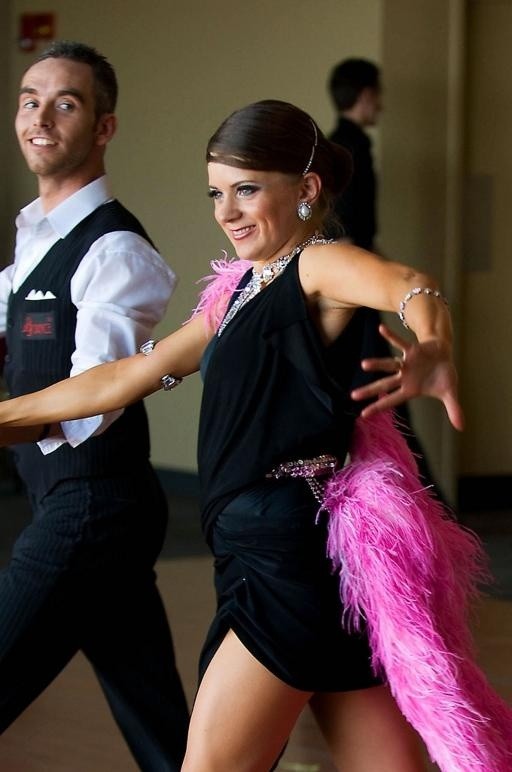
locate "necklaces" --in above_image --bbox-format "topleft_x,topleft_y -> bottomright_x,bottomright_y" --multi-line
216,230 -> 337,339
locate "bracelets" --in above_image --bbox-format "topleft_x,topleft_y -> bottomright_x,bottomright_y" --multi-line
398,286 -> 450,331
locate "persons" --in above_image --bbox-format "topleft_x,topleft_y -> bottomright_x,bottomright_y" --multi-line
1,99 -> 467,772
328,58 -> 444,509
0,41 -> 190,772
